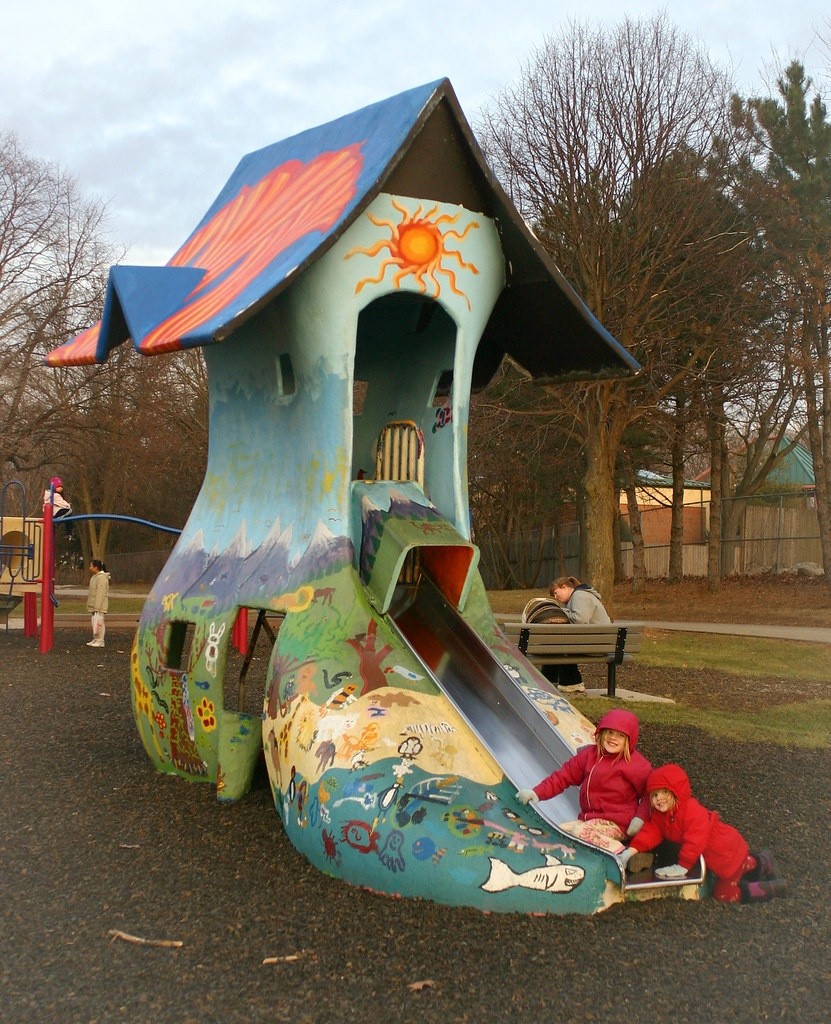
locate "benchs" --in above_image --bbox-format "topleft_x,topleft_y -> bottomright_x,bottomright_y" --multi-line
503,622 -> 645,699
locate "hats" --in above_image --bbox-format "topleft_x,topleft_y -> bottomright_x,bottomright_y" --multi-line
50,477 -> 63,490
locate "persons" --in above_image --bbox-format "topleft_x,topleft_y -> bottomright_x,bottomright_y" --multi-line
516,709 -> 660,873
86,560 -> 112,647
42,476 -> 77,542
541,576 -> 611,692
617,764 -> 790,905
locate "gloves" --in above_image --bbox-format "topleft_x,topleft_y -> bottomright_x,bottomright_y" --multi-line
626,816 -> 644,836
515,788 -> 540,806
618,849 -> 634,868
654,864 -> 688,877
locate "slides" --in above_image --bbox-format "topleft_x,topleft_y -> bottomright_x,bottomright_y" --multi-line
263,574 -> 711,920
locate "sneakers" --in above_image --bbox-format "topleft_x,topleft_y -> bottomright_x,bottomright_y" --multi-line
86,638 -> 97,646
557,681 -> 585,693
90,638 -> 105,647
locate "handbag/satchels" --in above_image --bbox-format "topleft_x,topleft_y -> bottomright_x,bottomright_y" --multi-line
91,611 -> 103,635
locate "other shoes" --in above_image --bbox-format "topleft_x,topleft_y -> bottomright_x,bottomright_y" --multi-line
64,535 -> 77,541
756,850 -> 782,880
759,877 -> 788,899
628,852 -> 654,872
